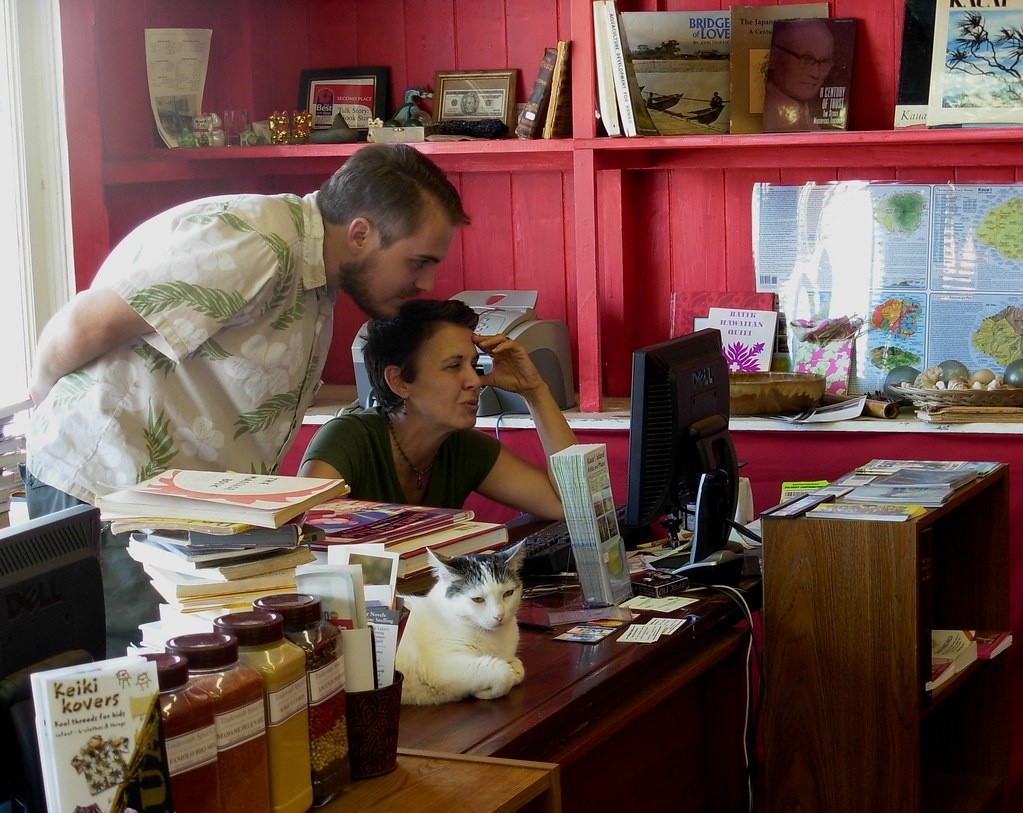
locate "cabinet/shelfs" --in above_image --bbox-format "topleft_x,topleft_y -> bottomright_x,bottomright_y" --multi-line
761,462 -> 1010,813
59,0 -> 1023,813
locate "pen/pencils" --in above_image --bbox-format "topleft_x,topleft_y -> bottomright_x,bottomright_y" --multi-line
517,621 -> 557,631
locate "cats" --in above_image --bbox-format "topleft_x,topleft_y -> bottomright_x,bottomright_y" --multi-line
392,536 -> 531,707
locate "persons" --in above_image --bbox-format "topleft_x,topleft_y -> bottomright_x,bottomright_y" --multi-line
710,92 -> 723,109
296,295 -> 581,520
646,91 -> 659,110
17,142 -> 471,660
761,20 -> 837,133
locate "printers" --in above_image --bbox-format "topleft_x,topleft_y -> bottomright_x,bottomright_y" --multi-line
349,290 -> 577,419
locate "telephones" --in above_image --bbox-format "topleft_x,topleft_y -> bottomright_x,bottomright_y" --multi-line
671,469 -> 762,589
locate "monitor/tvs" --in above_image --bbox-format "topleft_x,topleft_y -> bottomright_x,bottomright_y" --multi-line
625,327 -> 746,589
0,502 -> 106,813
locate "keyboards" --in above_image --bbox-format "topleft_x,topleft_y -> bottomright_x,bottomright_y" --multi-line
512,503 -> 653,577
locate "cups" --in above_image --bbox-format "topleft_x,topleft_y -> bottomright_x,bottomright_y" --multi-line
346,670 -> 405,781
223,109 -> 247,147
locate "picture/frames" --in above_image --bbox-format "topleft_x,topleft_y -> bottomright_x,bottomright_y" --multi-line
298,66 -> 389,141
430,69 -> 518,139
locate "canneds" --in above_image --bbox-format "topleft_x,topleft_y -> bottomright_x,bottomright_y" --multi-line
115,591 -> 350,813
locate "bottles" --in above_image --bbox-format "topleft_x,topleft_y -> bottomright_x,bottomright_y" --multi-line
136,592 -> 345,813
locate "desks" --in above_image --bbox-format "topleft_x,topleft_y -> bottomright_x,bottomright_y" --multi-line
397,522 -> 763,813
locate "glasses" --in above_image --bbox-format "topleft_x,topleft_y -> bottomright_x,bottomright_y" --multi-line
771,44 -> 838,72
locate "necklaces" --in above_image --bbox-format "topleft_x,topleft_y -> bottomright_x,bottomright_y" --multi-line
386,412 -> 442,490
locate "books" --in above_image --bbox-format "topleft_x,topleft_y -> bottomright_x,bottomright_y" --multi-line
93,466 -> 509,659
894,0 -> 1023,131
806,459 -> 1001,522
932,628 -> 1013,690
592,0 -> 860,138
29,653 -> 175,813
669,292 -> 777,372
516,39 -> 573,139
919,527 -> 933,707
